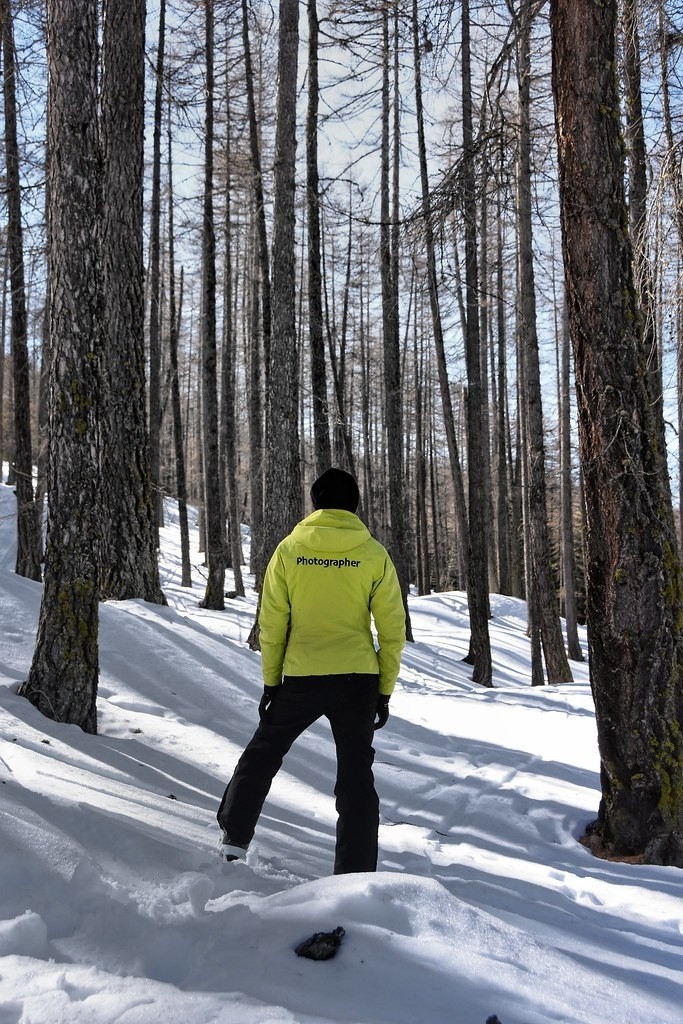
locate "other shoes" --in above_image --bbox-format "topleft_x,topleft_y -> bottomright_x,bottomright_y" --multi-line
218,830 -> 247,863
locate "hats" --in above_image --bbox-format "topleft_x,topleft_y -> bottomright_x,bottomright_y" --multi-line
310,467 -> 359,514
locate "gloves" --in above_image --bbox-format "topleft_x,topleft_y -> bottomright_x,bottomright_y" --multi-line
374,694 -> 390,731
258,680 -> 282,726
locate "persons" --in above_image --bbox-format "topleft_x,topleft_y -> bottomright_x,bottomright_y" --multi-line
216,467 -> 406,874
